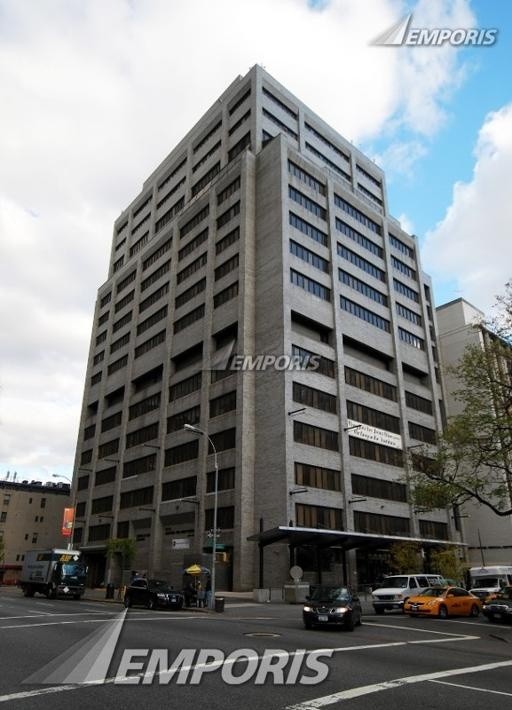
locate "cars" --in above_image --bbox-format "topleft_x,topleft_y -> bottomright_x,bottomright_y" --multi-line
372,565 -> 511,624
124,577 -> 184,614
302,585 -> 363,632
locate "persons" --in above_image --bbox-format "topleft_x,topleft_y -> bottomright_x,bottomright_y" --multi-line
181,578 -> 211,608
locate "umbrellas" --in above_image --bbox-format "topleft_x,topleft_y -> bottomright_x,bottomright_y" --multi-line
181,563 -> 211,590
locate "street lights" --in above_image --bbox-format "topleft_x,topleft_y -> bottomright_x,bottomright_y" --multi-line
52,473 -> 77,549
183,424 -> 219,612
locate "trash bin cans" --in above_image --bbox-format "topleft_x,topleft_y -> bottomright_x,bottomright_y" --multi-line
215,595 -> 225,612
106,583 -> 114,599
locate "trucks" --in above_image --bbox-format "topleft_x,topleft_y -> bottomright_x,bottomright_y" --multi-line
19,547 -> 89,601
